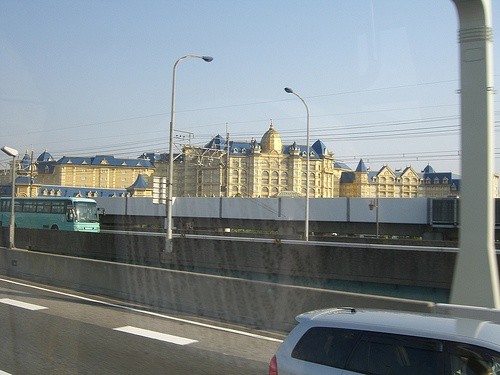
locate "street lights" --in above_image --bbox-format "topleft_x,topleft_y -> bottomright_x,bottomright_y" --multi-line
284,87 -> 311,242
165,55 -> 214,253
0,146 -> 18,248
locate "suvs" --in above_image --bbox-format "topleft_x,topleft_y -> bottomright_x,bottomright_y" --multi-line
268,306 -> 500,375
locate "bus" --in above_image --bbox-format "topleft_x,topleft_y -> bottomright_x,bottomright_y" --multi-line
0,197 -> 100,233
